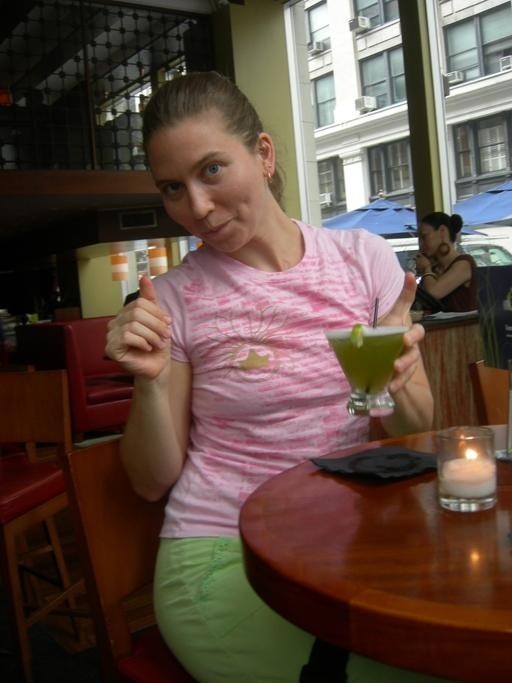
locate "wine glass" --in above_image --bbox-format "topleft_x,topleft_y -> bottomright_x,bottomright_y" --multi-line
321,318 -> 411,418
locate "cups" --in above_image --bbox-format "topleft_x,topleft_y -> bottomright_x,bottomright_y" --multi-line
438,513 -> 500,590
434,424 -> 497,512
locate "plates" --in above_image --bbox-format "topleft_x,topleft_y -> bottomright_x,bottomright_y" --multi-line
302,443 -> 434,481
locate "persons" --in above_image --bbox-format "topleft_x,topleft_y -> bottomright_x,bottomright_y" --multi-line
411,212 -> 483,313
103,70 -> 440,682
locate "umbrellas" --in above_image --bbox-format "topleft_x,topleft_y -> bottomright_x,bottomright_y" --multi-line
321,176 -> 512,237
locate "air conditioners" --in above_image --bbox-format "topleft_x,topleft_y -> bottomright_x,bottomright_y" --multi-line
348,16 -> 370,33
445,71 -> 464,85
307,40 -> 323,54
320,192 -> 331,208
499,55 -> 512,72
354,96 -> 377,113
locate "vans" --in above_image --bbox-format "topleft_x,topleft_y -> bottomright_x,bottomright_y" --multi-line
387,222 -> 512,284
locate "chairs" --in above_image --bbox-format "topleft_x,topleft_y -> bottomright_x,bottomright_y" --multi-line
13,315 -> 135,433
0,368 -> 97,682
66,431 -> 204,683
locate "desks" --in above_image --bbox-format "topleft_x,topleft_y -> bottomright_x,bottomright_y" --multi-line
366,309 -> 490,442
237,423 -> 512,683
